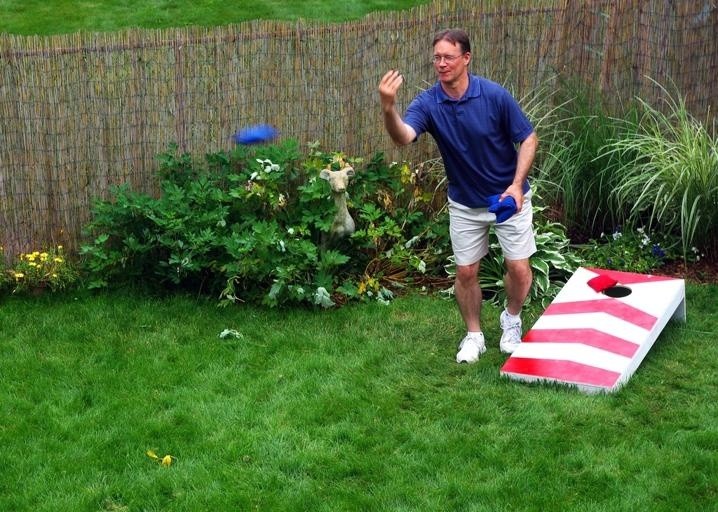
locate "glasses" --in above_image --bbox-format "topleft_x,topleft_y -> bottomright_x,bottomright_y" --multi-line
432,55 -> 462,63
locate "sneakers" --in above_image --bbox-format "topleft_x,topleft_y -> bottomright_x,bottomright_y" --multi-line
456,332 -> 487,364
499,309 -> 522,353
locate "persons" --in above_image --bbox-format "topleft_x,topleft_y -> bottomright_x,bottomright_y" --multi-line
379,28 -> 538,363
